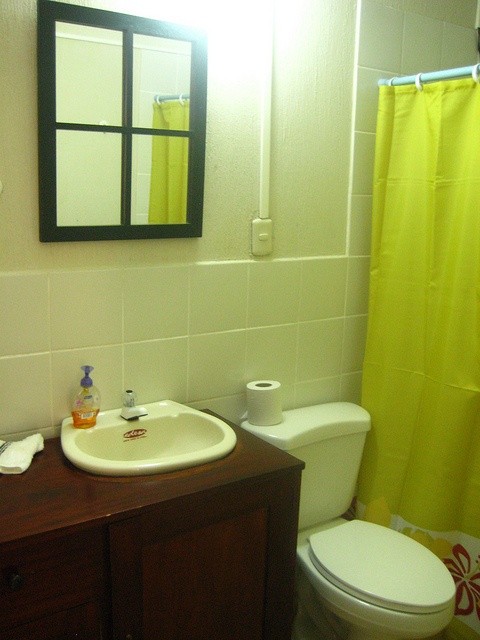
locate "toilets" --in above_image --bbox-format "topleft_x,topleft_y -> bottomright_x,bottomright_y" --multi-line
240,401 -> 461,640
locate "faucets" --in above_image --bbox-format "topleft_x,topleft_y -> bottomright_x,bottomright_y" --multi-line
120,389 -> 148,420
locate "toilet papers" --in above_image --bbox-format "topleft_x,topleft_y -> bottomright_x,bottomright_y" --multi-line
246,380 -> 284,428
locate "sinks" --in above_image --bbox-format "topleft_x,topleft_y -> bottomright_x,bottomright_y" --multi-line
69,411 -> 230,468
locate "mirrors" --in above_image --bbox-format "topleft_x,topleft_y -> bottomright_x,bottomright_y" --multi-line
132,32 -> 191,132
130,133 -> 189,225
55,129 -> 122,227
55,21 -> 123,128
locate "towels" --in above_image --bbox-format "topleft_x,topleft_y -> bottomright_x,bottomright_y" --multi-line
0,432 -> 46,474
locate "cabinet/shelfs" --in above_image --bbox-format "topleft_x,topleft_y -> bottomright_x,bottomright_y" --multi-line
0,408 -> 306,640
